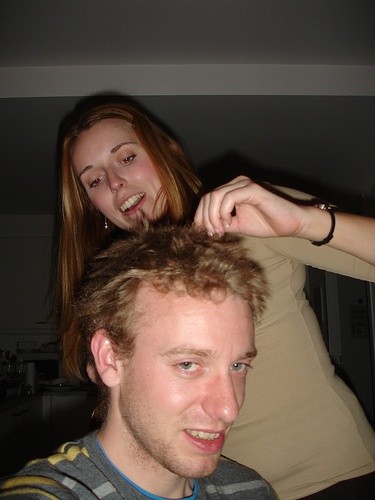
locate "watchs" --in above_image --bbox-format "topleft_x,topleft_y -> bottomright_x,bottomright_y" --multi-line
309,201 -> 336,247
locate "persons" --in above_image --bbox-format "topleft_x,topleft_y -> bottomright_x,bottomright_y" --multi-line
50,100 -> 375,500
0,219 -> 282,500
